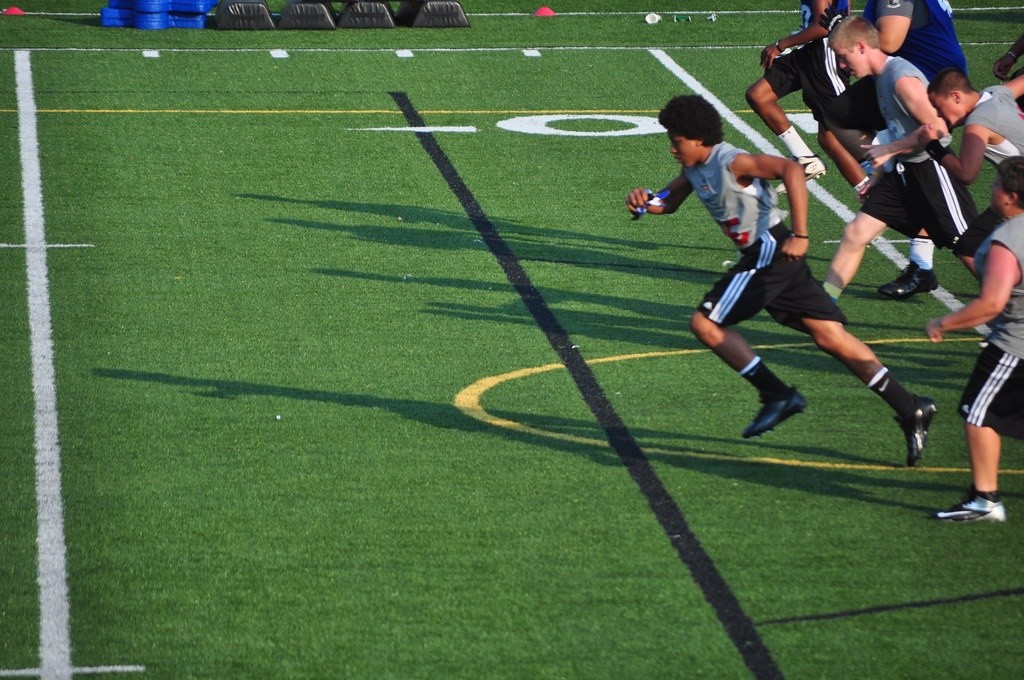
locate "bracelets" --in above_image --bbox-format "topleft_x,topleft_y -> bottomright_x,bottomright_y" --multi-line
1006,51 -> 1019,62
926,140 -> 956,164
788,231 -> 809,240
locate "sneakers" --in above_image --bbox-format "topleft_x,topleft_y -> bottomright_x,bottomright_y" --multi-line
774,152 -> 827,197
893,392 -> 938,466
936,484 -> 1008,522
877,260 -> 939,300
741,383 -> 807,440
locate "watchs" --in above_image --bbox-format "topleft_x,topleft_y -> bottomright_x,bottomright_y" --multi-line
775,40 -> 783,54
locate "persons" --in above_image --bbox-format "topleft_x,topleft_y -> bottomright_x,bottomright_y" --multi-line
745,0 -> 1024,299
626,94 -> 938,466
926,157 -> 1024,522
